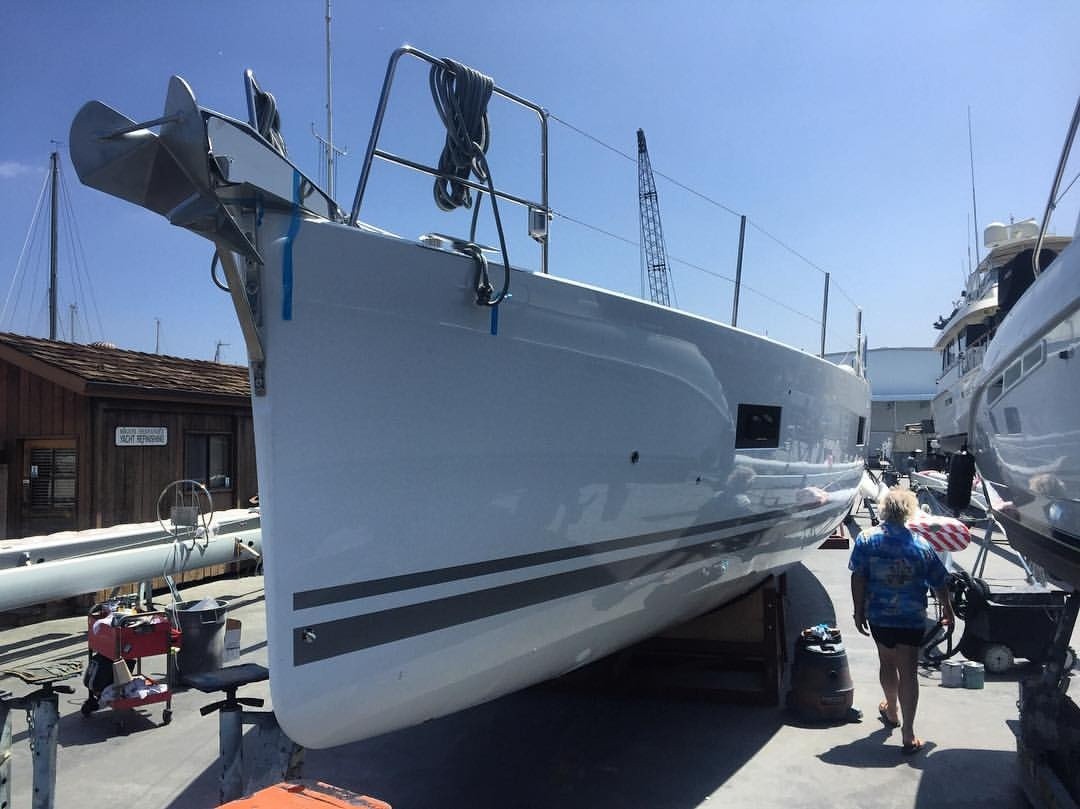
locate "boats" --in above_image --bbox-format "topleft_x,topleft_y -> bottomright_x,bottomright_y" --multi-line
69,41 -> 872,749
967,96 -> 1080,552
931,216 -> 1073,450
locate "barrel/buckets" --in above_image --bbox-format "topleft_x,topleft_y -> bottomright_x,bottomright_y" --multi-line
941,659 -> 985,689
165,600 -> 229,690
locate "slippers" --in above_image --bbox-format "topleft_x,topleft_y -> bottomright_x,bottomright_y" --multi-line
903,739 -> 925,755
879,702 -> 901,728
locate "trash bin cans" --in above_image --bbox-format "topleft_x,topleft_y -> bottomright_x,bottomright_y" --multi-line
165,599 -> 230,686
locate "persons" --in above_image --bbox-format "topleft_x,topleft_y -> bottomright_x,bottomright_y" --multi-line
848,486 -> 954,754
1029,474 -> 1080,550
653,465 -> 755,579
